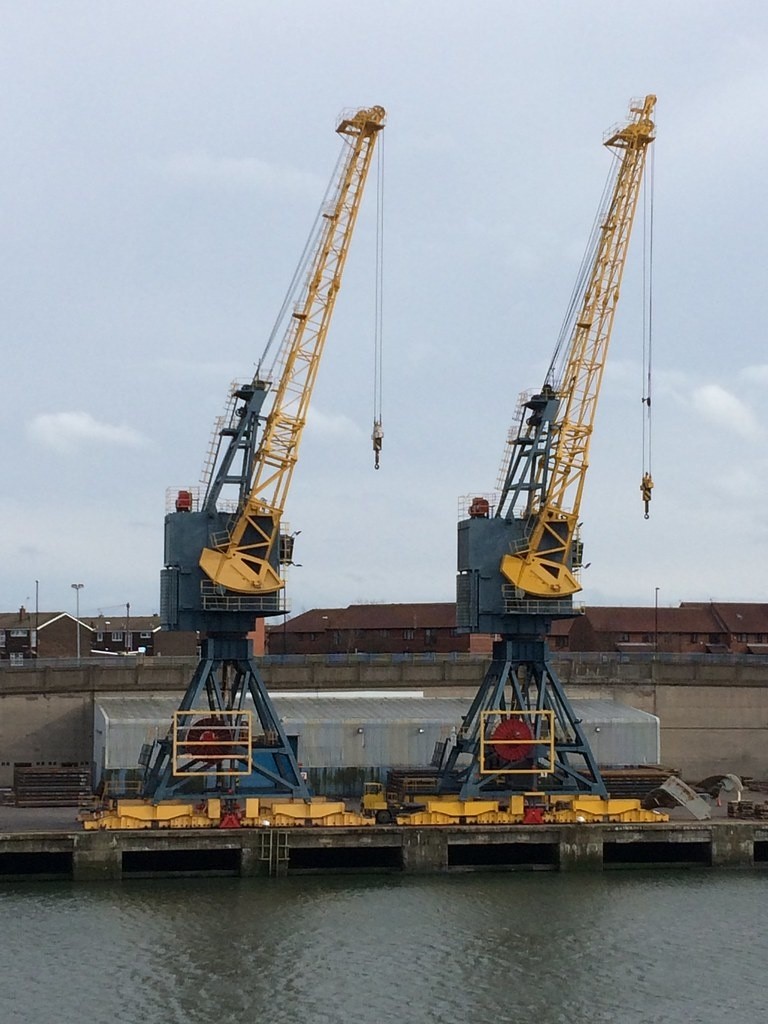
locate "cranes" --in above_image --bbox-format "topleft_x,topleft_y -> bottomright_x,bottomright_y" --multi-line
75,105 -> 389,831
360,92 -> 669,828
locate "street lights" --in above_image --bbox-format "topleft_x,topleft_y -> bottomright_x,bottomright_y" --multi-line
655,587 -> 660,654
71,583 -> 85,658
